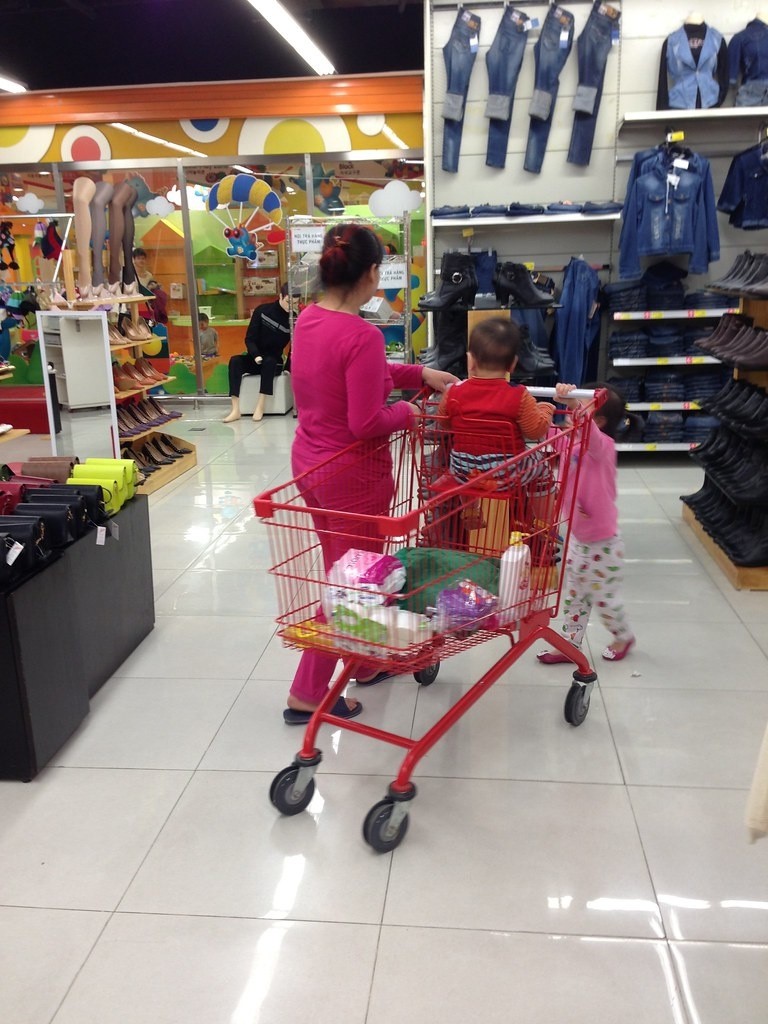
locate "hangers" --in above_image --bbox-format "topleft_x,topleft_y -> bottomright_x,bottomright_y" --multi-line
659,128 -> 694,174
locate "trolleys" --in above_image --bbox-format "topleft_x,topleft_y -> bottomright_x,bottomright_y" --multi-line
254,385 -> 607,854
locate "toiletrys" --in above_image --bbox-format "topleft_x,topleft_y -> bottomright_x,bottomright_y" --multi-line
495,530 -> 533,628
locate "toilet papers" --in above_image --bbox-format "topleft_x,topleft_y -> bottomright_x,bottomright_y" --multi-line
334,602 -> 434,658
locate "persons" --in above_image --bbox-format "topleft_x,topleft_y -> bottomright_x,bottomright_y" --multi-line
283,224 -> 462,727
133,249 -> 159,290
536,383 -> 645,664
436,319 -> 565,543
72,177 -> 139,298
222,282 -> 301,423
198,313 -> 218,357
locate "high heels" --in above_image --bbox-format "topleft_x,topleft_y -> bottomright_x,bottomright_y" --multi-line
415,251 -> 555,442
108,316 -> 193,487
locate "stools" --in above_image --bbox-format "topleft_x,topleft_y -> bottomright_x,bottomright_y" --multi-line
238,372 -> 295,417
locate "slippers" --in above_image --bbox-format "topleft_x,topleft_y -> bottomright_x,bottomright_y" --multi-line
283,695 -> 362,726
356,656 -> 414,687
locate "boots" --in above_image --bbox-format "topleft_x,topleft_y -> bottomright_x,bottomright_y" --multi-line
679,249 -> 768,566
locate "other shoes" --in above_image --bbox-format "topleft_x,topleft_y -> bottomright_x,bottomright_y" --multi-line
538,649 -> 572,664
602,636 -> 635,660
461,507 -> 486,529
529,519 -> 564,544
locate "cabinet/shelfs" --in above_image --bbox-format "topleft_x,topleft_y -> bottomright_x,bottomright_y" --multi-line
681,289 -> 768,590
413,209 -> 625,313
56,294 -> 198,495
604,308 -> 741,453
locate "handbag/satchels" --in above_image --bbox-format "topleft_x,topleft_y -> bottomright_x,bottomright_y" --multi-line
0,455 -> 138,596
